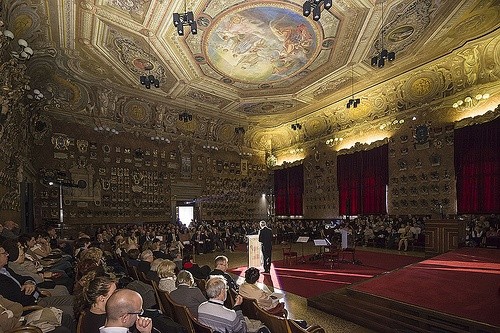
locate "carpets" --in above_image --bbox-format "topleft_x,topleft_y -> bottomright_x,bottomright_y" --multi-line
226,250 -> 424,298
350,247 -> 500,328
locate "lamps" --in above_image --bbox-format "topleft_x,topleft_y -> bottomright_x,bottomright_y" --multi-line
303,0 -> 332,21
173,0 -> 197,36
0,20 -> 34,63
371,3 -> 395,68
140,36 -> 159,89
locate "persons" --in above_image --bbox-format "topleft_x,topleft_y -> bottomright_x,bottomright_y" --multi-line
259,220 -> 273,273
0,214 -> 500,333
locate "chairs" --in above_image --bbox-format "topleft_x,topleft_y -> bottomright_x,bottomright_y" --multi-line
3,217 -> 500,333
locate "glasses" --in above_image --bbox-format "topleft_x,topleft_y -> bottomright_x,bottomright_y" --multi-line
119,310 -> 144,318
0,251 -> 7,254
